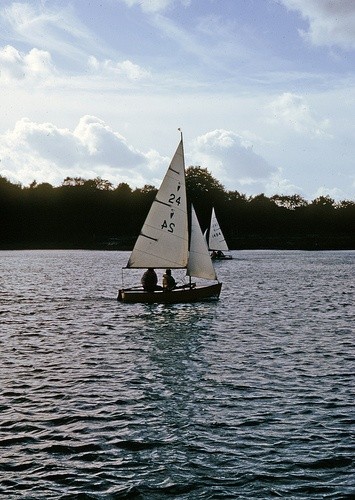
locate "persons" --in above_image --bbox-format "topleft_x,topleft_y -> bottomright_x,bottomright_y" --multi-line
211,250 -> 225,258
141,268 -> 163,294
162,270 -> 176,294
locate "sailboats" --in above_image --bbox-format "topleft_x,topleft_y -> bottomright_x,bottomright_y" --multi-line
115,128 -> 223,303
203,206 -> 233,261
